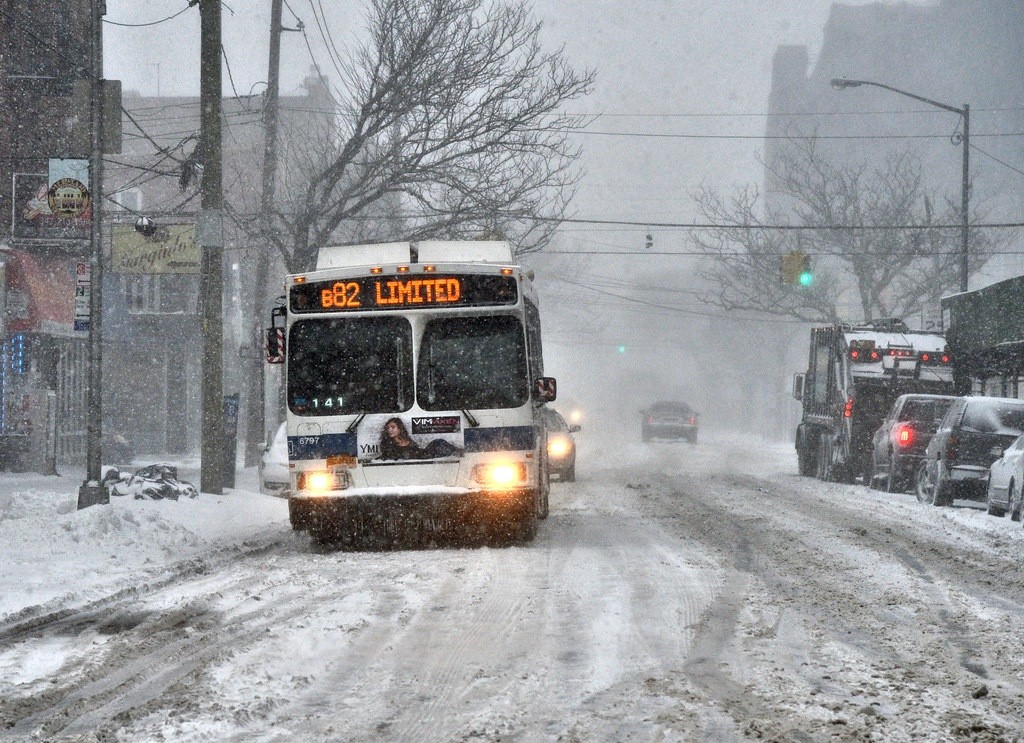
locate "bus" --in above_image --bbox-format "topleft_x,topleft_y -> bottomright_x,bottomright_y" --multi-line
264,238 -> 557,547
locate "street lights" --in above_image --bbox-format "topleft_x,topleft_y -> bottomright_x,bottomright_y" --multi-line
831,77 -> 972,295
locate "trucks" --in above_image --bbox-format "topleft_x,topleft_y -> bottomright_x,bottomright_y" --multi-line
794,320 -> 1024,489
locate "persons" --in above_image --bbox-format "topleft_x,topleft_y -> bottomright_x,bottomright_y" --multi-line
380,417 -> 464,460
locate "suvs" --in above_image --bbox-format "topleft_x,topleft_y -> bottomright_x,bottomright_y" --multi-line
868,393 -> 959,495
913,394 -> 1024,508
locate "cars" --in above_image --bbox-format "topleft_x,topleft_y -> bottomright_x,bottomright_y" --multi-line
639,400 -> 702,446
542,407 -> 582,483
257,420 -> 292,498
986,433 -> 1024,522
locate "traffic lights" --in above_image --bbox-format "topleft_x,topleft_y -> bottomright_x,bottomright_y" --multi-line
793,252 -> 813,288
778,254 -> 796,289
